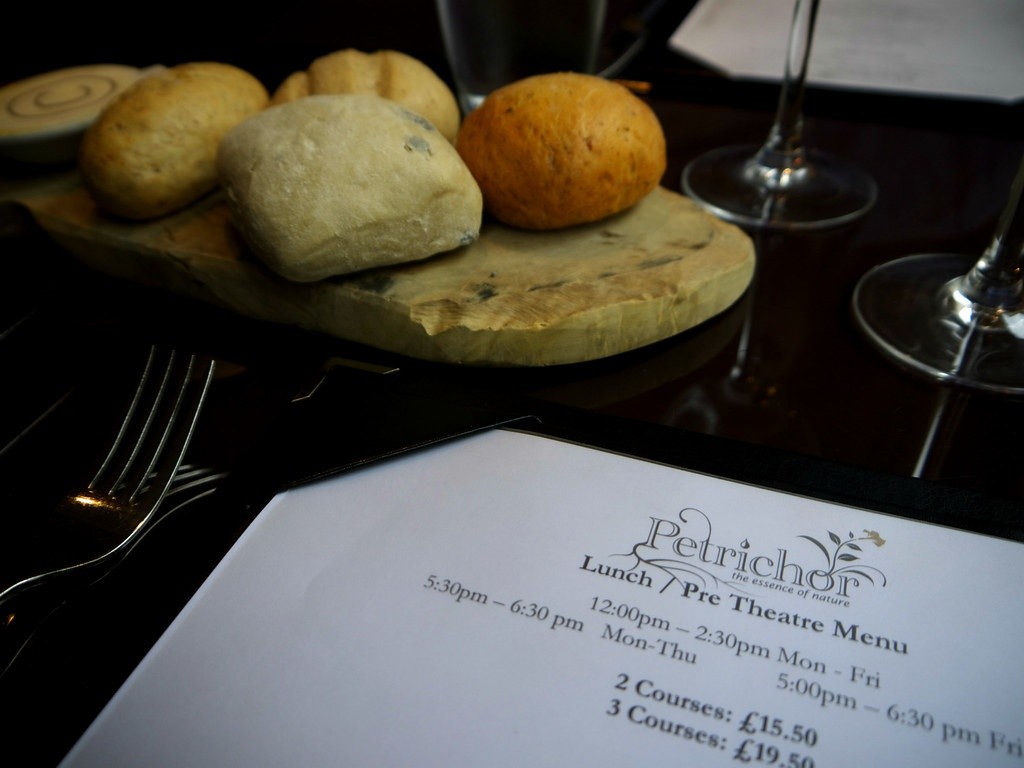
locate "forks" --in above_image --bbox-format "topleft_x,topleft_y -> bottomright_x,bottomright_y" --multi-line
0,466 -> 227,676
0,339 -> 217,620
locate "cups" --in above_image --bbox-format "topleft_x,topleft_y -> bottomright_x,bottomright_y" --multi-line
432,1 -> 607,116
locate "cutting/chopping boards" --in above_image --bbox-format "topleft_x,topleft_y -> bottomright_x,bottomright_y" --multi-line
0,101 -> 759,364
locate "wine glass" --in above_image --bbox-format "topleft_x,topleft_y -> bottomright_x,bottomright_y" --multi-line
680,0 -> 886,230
850,157 -> 1024,395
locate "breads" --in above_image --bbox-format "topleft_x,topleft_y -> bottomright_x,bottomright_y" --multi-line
0,50 -> 666,286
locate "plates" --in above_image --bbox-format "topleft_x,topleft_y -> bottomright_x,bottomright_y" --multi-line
662,0 -> 1024,106
